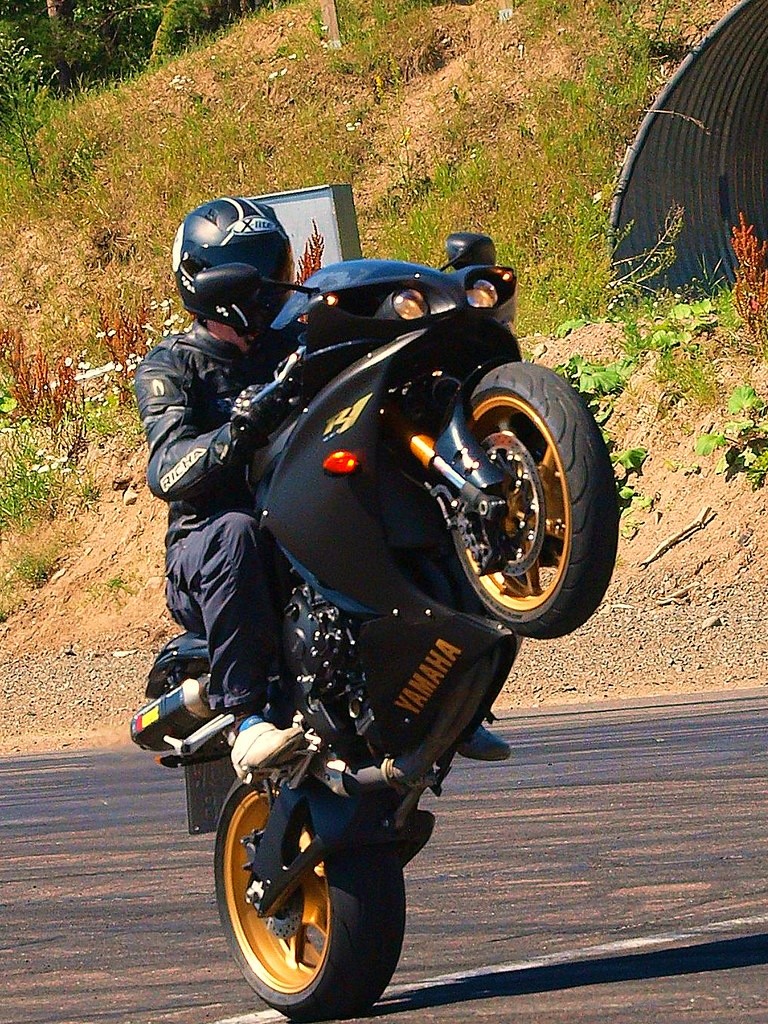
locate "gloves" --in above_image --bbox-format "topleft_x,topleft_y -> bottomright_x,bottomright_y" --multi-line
230,384 -> 291,440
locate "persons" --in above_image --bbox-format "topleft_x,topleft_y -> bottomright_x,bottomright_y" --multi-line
134,197 -> 511,781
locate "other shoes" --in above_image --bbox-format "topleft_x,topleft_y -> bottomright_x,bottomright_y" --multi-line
230,721 -> 303,779
457,725 -> 510,762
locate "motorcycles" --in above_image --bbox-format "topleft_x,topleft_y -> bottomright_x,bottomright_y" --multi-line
126,229 -> 624,1024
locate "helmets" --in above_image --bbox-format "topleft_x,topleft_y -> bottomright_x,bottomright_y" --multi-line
172,197 -> 293,325
